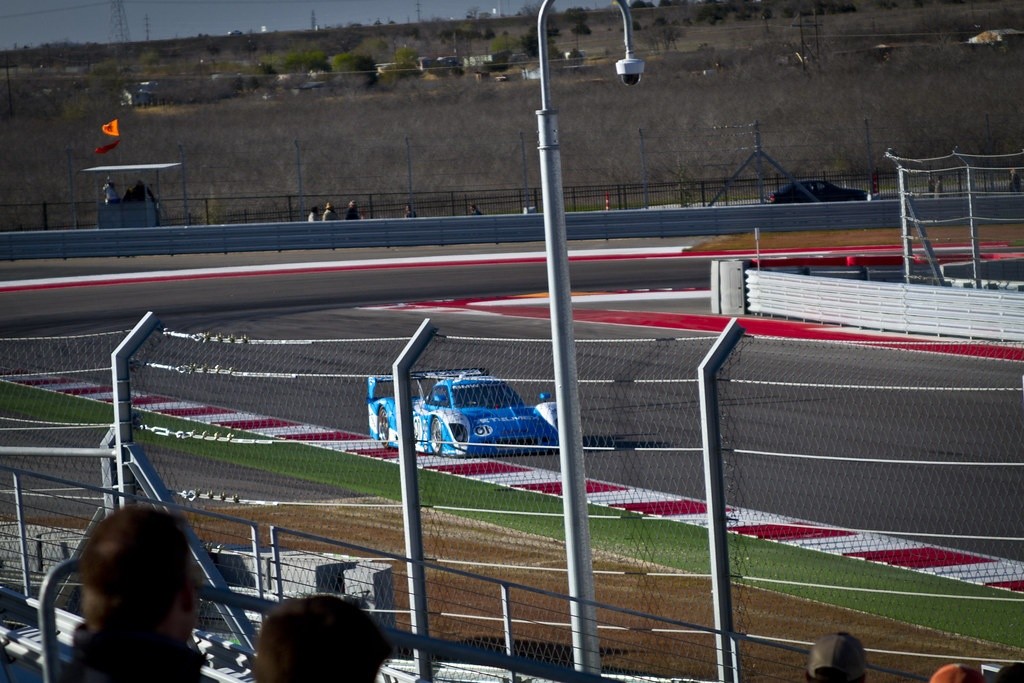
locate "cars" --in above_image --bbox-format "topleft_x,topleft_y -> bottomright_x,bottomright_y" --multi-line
769,179 -> 869,203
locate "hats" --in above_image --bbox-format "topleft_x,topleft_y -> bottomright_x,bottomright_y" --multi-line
929,663 -> 987,683
806,632 -> 865,683
349,200 -> 357,206
326,203 -> 334,208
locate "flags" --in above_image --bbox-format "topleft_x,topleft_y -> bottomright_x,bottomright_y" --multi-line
95,140 -> 119,153
102,119 -> 119,136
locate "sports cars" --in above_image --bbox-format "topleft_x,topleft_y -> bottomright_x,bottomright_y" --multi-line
367,367 -> 559,457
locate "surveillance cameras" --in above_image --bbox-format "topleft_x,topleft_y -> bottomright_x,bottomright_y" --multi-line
615,58 -> 645,85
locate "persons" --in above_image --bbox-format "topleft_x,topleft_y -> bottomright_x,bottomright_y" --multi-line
102,182 -> 120,204
470,205 -> 482,215
344,200 -> 359,219
992,662 -> 1024,683
927,176 -> 934,198
1010,170 -> 1019,192
929,664 -> 987,683
938,178 -> 943,198
307,206 -> 319,221
67,506 -> 206,683
323,202 -> 337,220
805,631 -> 867,683
255,595 -> 393,683
404,205 -> 416,217
123,180 -> 154,201
